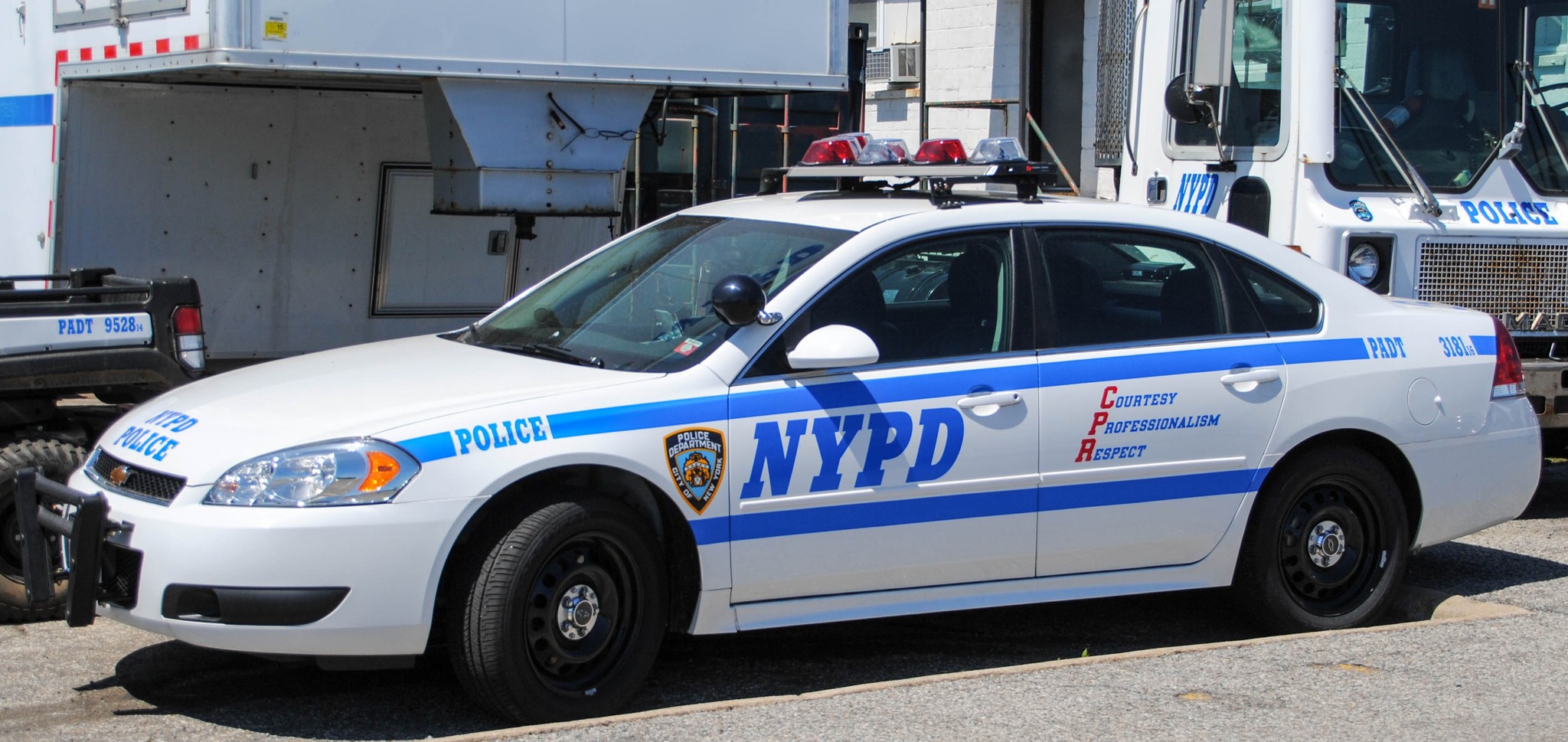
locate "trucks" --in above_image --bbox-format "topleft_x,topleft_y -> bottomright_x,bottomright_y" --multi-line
1094,2 -> 1568,470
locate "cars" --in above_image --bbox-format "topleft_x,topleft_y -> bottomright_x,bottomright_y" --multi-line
17,134 -> 1542,729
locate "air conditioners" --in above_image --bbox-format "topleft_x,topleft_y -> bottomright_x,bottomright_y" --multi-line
867,44 -> 921,83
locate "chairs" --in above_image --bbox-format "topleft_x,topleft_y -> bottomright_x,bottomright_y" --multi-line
1158,269 -> 1207,326
1050,252 -> 1107,309
910,251 -> 998,354
831,269 -> 900,356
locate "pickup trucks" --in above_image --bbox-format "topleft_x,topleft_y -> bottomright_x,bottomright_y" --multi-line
0,266 -> 212,626
